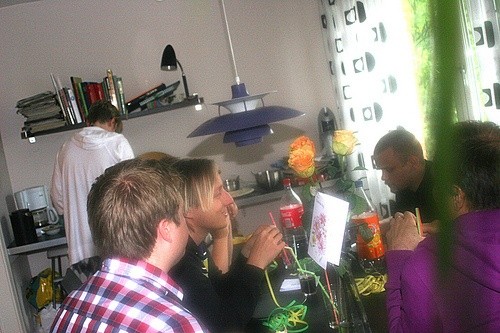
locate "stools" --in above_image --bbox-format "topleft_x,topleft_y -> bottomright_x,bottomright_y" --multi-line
44,241 -> 76,309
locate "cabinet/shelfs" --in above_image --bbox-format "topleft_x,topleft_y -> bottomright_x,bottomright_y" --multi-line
5,172 -> 381,333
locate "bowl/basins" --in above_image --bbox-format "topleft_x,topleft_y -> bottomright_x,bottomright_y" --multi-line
255,169 -> 281,190
41,225 -> 60,235
222,179 -> 239,192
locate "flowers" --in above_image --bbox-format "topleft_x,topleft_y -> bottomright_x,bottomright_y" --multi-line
286,128 -> 361,210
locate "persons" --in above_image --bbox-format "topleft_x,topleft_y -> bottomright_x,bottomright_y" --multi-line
50,99 -> 136,263
373,120 -> 500,333
48,153 -> 286,333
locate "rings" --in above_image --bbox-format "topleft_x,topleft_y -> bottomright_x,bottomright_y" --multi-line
396,215 -> 403,219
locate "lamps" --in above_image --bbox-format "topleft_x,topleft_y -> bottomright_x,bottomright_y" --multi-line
185,0 -> 308,154
158,42 -> 199,106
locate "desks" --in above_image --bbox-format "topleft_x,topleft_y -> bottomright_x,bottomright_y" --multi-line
225,222 -> 397,333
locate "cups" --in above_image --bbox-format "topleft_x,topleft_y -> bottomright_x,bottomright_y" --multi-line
298,271 -> 317,301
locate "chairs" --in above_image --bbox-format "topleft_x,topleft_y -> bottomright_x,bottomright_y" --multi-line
62,255 -> 106,296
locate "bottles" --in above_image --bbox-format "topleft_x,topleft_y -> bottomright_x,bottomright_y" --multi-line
10,209 -> 38,245
279,178 -> 309,260
349,181 -> 383,273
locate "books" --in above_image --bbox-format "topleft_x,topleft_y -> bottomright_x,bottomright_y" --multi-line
51,68 -> 181,126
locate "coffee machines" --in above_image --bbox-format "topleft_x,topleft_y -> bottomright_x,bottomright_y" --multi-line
15,186 -> 52,235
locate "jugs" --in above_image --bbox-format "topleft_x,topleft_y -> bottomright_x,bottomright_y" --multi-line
30,208 -> 58,228
